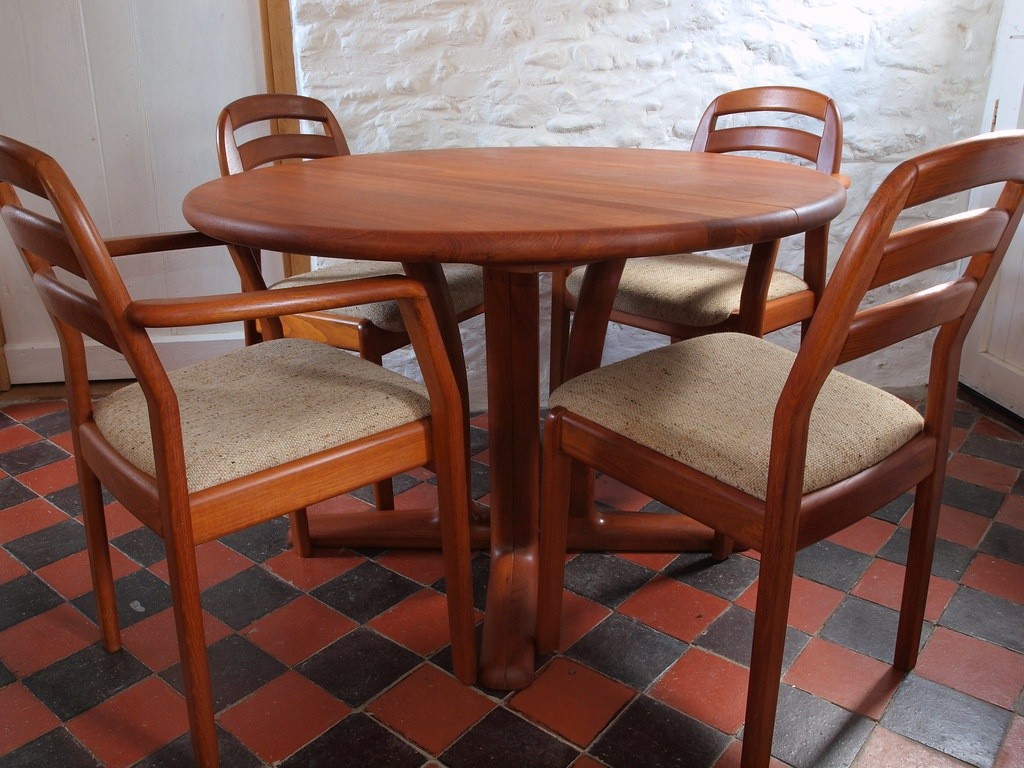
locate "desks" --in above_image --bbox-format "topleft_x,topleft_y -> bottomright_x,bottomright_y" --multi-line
182,146 -> 847,692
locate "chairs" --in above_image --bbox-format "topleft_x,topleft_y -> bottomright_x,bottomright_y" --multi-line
538,128 -> 1024,768
0,132 -> 477,768
217,93 -> 486,512
553,87 -> 842,391
